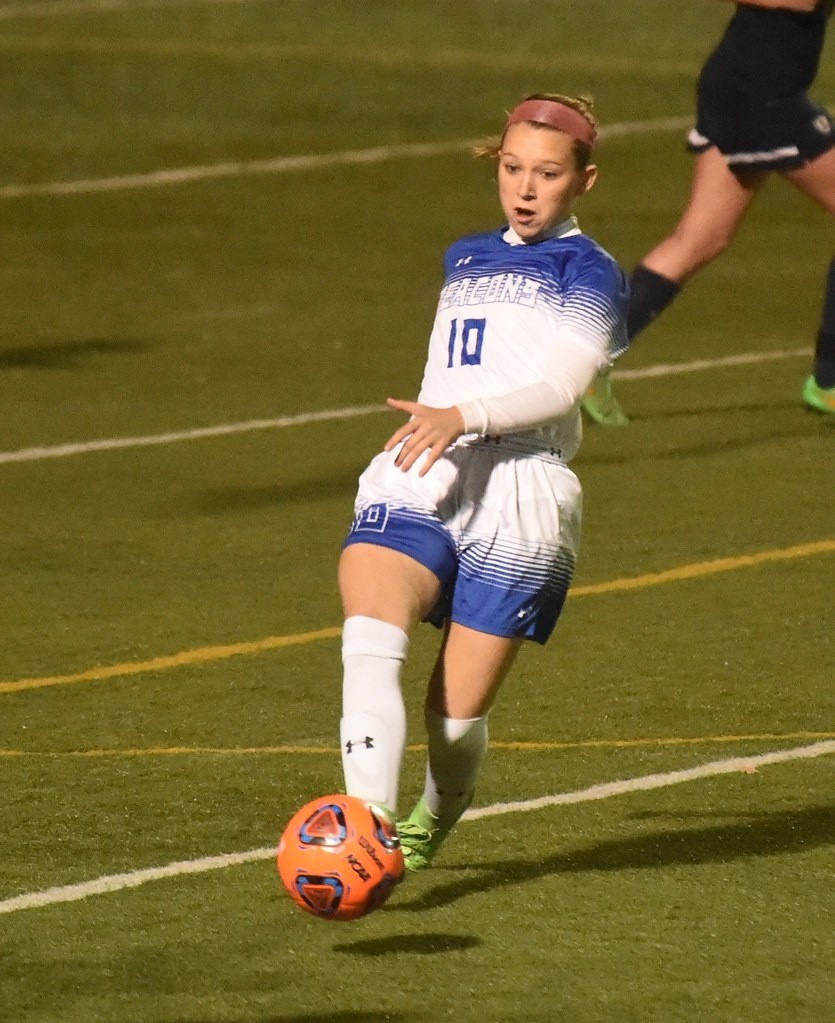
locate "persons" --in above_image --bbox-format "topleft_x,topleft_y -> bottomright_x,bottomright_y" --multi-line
339,90 -> 626,876
576,0 -> 835,425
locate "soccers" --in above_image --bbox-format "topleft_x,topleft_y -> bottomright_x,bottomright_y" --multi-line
275,793 -> 408,923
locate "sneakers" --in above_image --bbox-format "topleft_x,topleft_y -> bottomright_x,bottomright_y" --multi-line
583,366 -> 629,426
371,786 -> 476,875
801,374 -> 834,414
